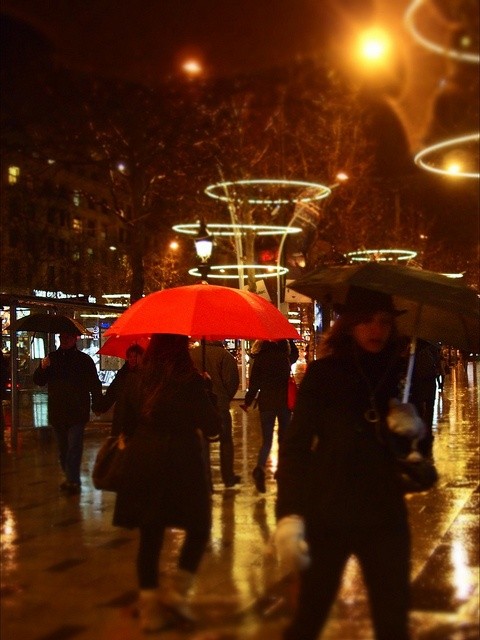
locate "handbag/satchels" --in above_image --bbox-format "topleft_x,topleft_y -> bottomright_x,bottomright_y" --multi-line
287,367 -> 297,411
92,438 -> 135,492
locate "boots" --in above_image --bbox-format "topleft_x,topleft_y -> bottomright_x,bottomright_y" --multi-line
139,590 -> 180,631
162,570 -> 198,625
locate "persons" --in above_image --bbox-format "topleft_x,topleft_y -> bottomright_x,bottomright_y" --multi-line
103,344 -> 144,438
275,285 -> 424,636
189,339 -> 240,486
33,332 -> 102,497
94,333 -> 221,585
240,339 -> 300,493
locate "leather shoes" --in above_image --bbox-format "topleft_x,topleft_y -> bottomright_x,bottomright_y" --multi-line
60,480 -> 81,489
224,473 -> 242,487
253,467 -> 267,493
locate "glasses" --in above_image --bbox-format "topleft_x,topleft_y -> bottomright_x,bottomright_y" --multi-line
59,335 -> 75,339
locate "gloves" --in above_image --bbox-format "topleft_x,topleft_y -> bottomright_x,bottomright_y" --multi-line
388,398 -> 425,437
265,515 -> 311,577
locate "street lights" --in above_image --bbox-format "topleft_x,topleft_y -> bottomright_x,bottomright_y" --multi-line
191,218 -> 214,375
277,171 -> 349,310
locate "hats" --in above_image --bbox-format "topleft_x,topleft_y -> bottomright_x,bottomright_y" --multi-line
333,286 -> 408,316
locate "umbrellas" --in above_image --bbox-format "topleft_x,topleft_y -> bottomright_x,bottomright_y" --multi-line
4,313 -> 94,357
94,336 -> 151,371
101,281 -> 304,372
285,262 -> 480,405
